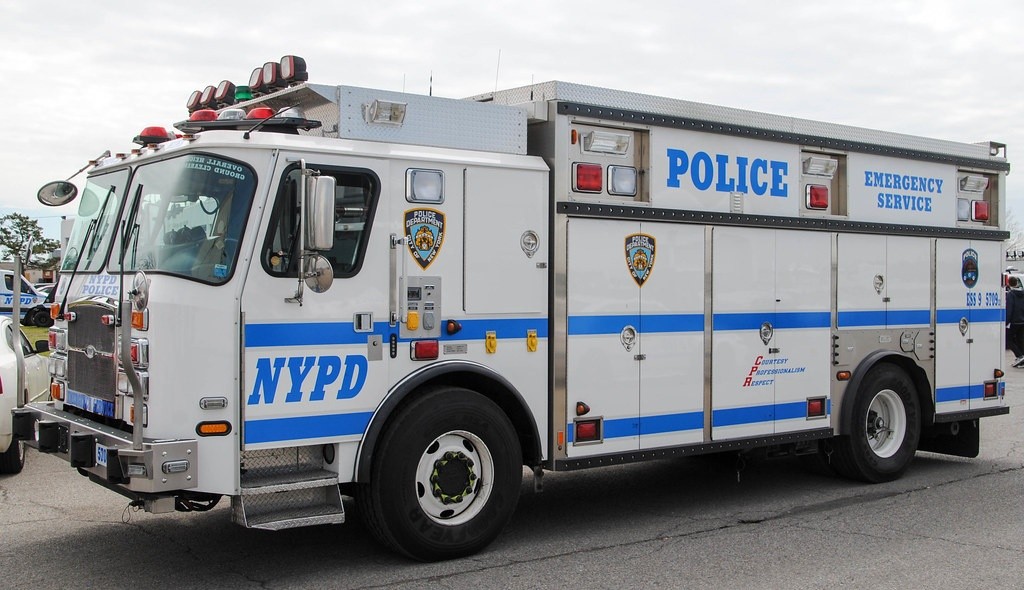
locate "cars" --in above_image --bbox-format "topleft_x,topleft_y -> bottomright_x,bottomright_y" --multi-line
35,282 -> 57,298
0,314 -> 49,475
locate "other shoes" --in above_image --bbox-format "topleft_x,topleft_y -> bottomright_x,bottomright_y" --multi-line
1012,355 -> 1024,367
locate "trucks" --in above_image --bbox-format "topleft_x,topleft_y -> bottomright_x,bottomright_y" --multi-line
0,269 -> 50,312
9,55 -> 1008,564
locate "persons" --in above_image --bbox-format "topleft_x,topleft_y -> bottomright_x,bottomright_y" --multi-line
1006,276 -> 1024,367
